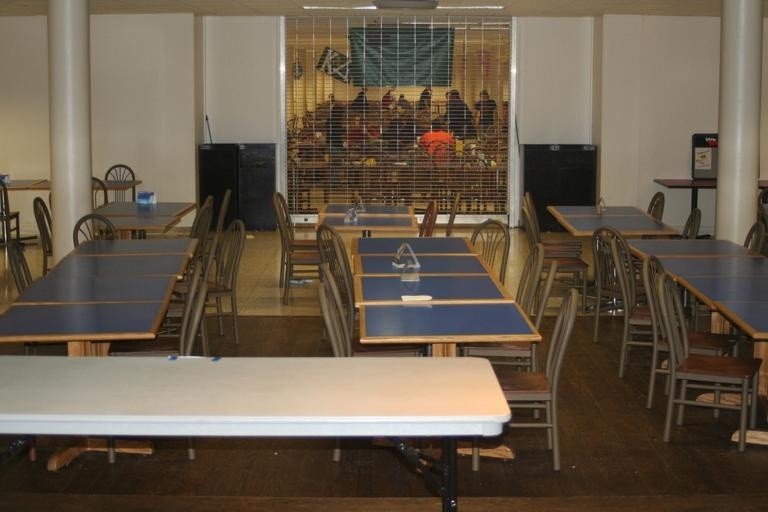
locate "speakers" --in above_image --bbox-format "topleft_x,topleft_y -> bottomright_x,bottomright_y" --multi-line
522,145 -> 561,232
562,145 -> 599,205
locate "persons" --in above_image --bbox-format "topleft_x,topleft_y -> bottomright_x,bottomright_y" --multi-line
442,91 -> 478,139
378,120 -> 415,154
418,86 -> 433,111
397,94 -> 411,108
352,93 -> 367,107
380,90 -> 396,109
472,90 -> 498,133
346,121 -> 376,146
328,94 -> 335,101
325,105 -> 343,189
418,118 -> 456,164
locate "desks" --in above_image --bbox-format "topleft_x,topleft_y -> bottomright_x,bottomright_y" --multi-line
36,253 -> 190,282
556,214 -> 680,237
318,204 -> 414,216
714,301 -> 768,448
28,179 -> 144,191
69,237 -> 200,259
92,201 -> 198,218
0,179 -> 47,190
618,238 -> 762,259
546,205 -> 650,216
352,276 -> 515,309
676,276 -> 766,407
359,301 -> 543,462
653,177 -> 767,212
0,355 -> 512,512
353,254 -> 497,276
315,215 -> 419,233
648,256 -> 768,282
350,236 -> 481,259
1,302 -> 171,472
93,214 -> 183,238
16,276 -> 177,304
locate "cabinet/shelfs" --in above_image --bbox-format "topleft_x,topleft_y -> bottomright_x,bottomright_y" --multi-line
519,143 -> 597,233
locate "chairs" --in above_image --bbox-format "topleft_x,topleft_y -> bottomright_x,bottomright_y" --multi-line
641,253 -> 744,419
459,258 -> 559,420
521,204 -> 589,316
91,176 -> 107,210
515,242 -> 545,319
156,234 -> 220,357
1,181 -> 20,244
33,196 -> 54,277
109,280 -> 209,461
610,236 -> 692,378
332,235 -> 355,340
288,98 -> 515,212
6,239 -> 35,295
273,192 -> 332,289
272,192 -> 336,306
315,222 -> 353,342
646,190 -> 673,221
656,272 -> 764,453
524,191 -> 583,245
318,282 -> 424,357
682,209 -> 703,240
418,200 -> 438,238
318,263 -> 421,352
215,190 -> 232,275
742,220 -> 766,255
471,287 -> 579,472
446,191 -> 462,236
590,225 -> 648,344
108,259 -> 204,357
470,218 -> 511,286
73,213 -> 119,250
521,195 -> 582,260
204,194 -> 214,206
190,203 -> 214,282
172,219 -> 246,345
104,164 -> 137,202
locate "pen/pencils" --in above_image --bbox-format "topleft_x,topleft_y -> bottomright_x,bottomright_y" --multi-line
168,355 -> 221,361
